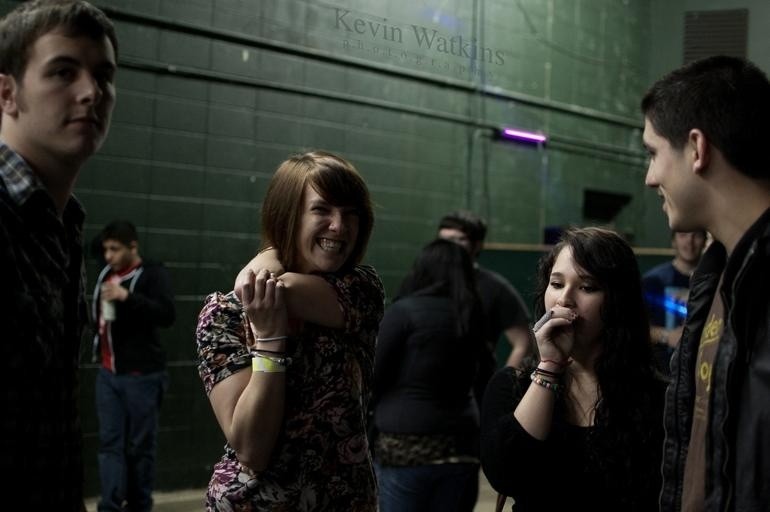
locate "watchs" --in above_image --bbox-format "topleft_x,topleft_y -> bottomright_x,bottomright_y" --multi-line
660,330 -> 669,344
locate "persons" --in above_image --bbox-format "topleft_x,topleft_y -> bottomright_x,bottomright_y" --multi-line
91,220 -> 176,512
641,54 -> 769,511
0,0 -> 119,512
370,239 -> 500,512
195,151 -> 386,512
435,211 -> 535,374
644,228 -> 708,366
478,226 -> 667,512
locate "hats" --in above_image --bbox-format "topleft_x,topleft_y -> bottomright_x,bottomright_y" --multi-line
437,209 -> 488,259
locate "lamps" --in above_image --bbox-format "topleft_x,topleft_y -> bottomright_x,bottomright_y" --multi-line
498,123 -> 550,147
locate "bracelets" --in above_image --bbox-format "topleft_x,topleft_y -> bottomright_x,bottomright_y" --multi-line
526,365 -> 567,392
251,337 -> 292,373
